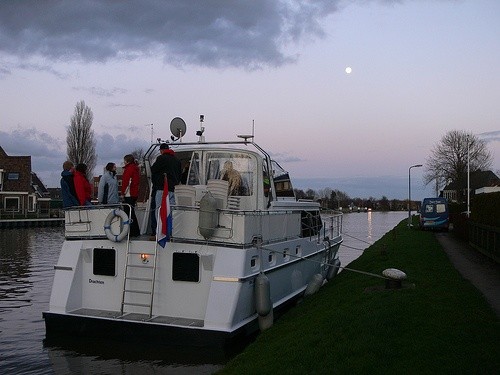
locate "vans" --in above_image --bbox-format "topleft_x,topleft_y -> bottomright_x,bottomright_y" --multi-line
419,197 -> 450,230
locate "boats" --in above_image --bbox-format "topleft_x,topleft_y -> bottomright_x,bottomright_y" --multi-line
42,115 -> 344,356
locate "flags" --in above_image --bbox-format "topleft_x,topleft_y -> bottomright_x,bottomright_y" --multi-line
157,176 -> 175,248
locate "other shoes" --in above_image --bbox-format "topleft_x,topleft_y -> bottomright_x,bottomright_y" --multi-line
149,235 -> 156,241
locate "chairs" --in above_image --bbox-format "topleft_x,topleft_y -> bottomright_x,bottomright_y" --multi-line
174,178 -> 240,213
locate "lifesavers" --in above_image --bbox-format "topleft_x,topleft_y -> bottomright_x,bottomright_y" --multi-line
103,210 -> 129,242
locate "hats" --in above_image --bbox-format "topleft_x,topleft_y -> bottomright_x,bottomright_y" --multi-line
158,143 -> 169,151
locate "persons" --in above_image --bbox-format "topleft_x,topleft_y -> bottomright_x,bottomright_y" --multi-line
60,161 -> 78,211
119,154 -> 141,238
73,163 -> 94,209
217,162 -> 241,196
97,163 -> 120,207
147,143 -> 181,240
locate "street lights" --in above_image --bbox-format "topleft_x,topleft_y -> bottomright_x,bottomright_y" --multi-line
406,164 -> 424,229
